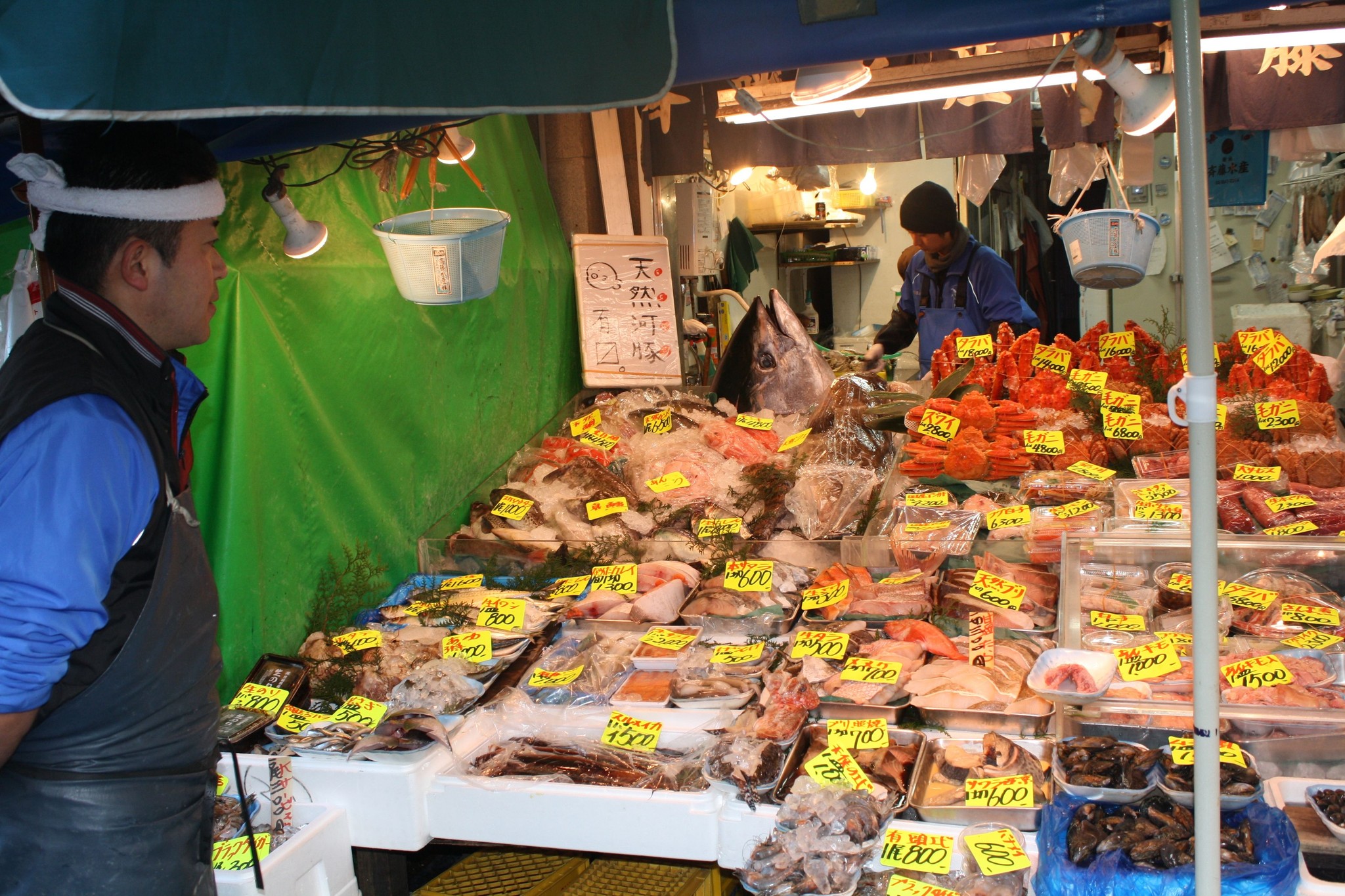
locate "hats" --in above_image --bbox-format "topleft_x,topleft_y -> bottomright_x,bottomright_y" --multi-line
900,181 -> 957,234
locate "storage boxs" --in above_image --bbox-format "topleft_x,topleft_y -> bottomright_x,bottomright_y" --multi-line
210,571 -> 1345,895
1229,301 -> 1313,353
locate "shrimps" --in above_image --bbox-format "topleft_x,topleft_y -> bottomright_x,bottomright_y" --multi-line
731,791 -> 899,896
213,791 -> 258,844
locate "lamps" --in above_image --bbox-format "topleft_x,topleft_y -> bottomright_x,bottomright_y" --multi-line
715,25 -> 1162,126
1071,28 -> 1177,136
437,121 -> 475,165
261,152 -> 328,259
789,57 -> 872,106
1165,0 -> 1345,54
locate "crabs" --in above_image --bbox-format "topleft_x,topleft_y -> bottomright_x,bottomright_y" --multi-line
897,325 -> 1337,484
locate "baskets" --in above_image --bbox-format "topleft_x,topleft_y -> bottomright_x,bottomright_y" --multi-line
1058,209 -> 1160,289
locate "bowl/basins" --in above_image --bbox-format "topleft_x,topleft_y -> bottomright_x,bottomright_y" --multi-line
1156,743 -> 1265,811
1305,780 -> 1344,844
1053,735 -> 1158,804
1026,648 -> 1118,706
1152,560 -> 1191,611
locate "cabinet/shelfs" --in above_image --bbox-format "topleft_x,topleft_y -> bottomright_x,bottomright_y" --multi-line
745,216 -> 881,280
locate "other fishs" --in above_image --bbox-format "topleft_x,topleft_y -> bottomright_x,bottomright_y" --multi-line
506,286 -> 896,483
239,484 -> 1343,791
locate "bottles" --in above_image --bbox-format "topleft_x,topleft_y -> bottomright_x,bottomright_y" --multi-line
875,195 -> 894,207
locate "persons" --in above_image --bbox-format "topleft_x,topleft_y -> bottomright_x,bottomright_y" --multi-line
1,125 -> 222,896
857,180 -> 1022,380
899,243 -> 1040,334
